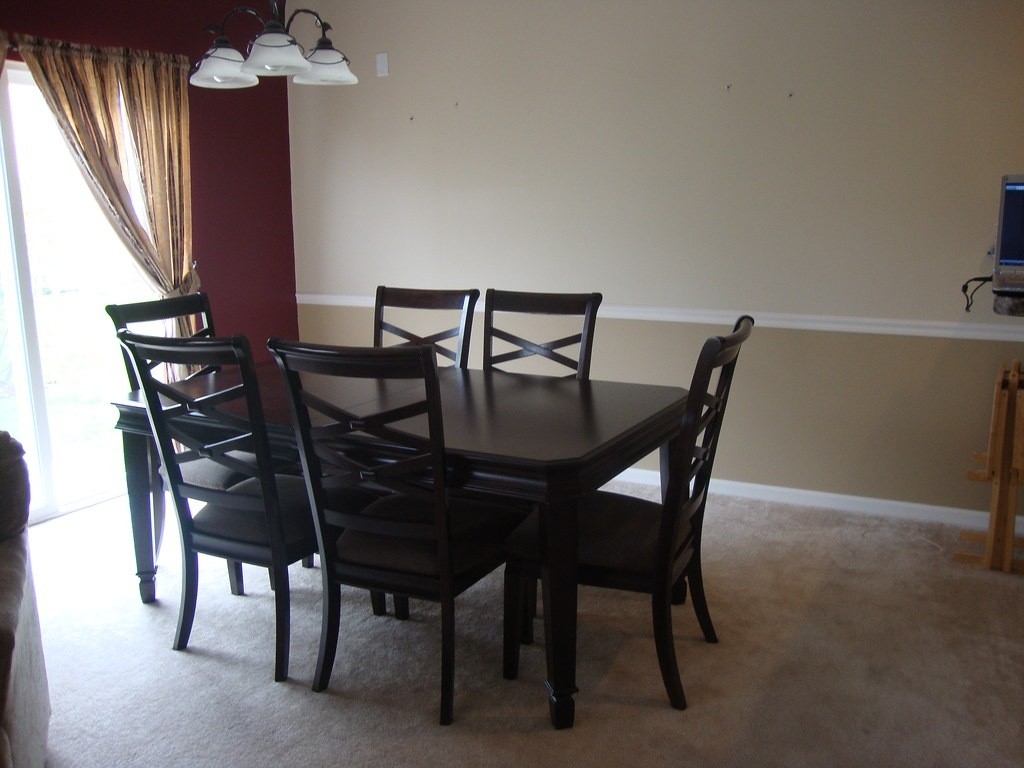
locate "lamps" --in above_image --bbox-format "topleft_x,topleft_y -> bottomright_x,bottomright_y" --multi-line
188,0 -> 359,90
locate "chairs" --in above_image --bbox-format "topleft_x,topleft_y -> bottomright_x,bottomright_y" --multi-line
116,327 -> 388,685
101,290 -> 314,595
485,314 -> 759,731
481,288 -> 603,377
266,337 -> 535,728
373,282 -> 480,367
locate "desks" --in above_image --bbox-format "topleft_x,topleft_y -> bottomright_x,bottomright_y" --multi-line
108,365 -> 694,729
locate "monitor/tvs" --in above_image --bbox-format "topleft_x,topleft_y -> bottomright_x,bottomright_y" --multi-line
993,175 -> 1024,296
